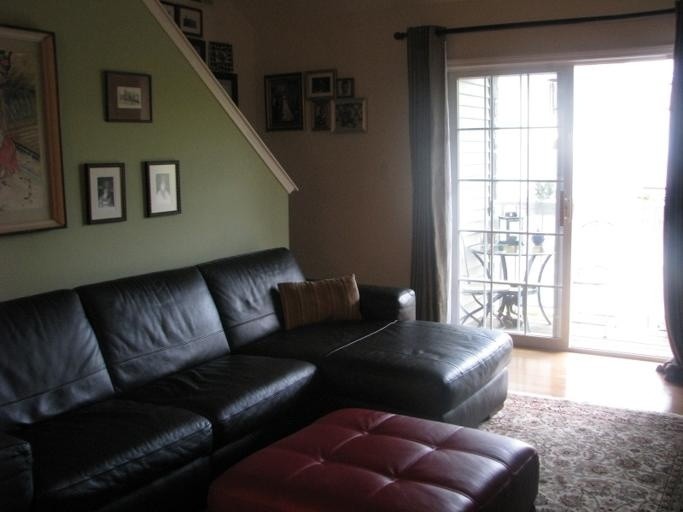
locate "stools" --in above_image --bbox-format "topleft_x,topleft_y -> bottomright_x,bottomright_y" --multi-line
209,408 -> 539,512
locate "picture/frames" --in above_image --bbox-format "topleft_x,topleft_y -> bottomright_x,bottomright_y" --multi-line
157,0 -> 238,107
105,70 -> 152,123
80,162 -> 126,224
0,22 -> 67,236
142,160 -> 181,217
263,68 -> 367,133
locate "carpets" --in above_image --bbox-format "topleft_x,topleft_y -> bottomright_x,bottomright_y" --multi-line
476,393 -> 683,512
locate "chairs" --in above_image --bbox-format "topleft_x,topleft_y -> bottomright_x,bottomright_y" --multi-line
460,234 -> 510,332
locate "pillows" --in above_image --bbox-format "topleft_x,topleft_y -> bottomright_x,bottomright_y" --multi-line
272,273 -> 364,333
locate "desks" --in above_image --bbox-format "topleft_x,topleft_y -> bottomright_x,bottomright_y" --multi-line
469,241 -> 552,333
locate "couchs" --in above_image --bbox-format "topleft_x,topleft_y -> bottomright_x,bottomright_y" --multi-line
318,319 -> 514,428
0,246 -> 417,512
